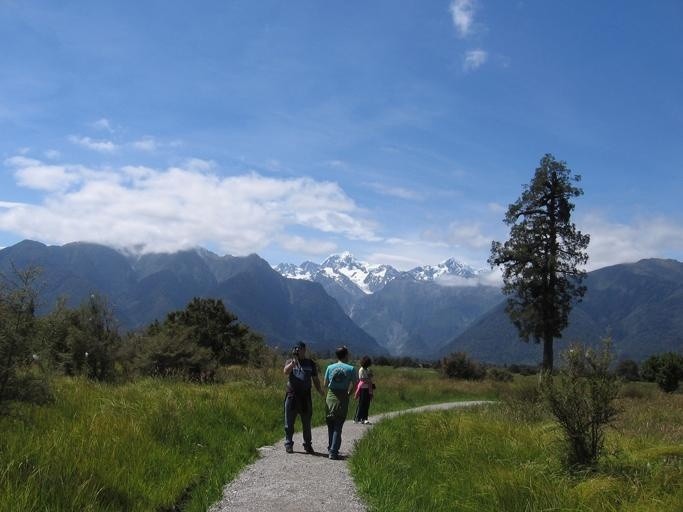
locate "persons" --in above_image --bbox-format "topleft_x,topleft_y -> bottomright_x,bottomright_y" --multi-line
281,340 -> 325,455
323,345 -> 358,461
356,356 -> 375,425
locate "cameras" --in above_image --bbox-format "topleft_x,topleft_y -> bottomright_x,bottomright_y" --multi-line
293,346 -> 302,359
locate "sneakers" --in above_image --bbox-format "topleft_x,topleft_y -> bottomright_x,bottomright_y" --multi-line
285,445 -> 339,461
353,418 -> 370,426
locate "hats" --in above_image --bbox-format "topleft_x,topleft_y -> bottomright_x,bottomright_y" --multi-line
292,340 -> 306,349
